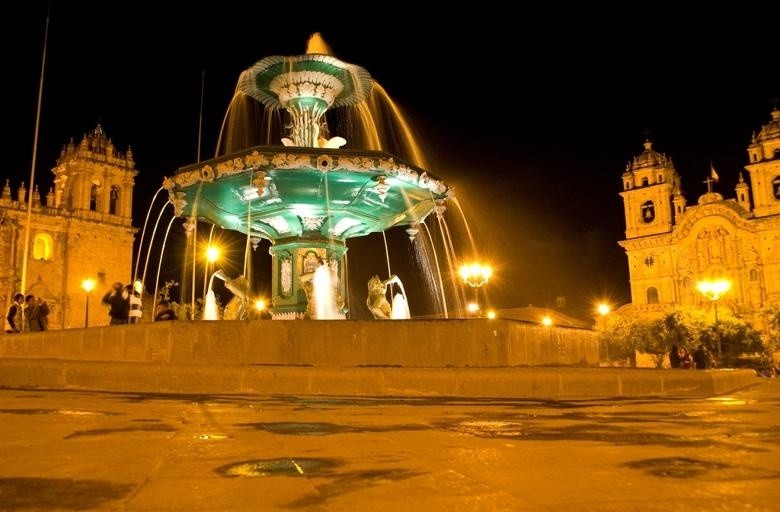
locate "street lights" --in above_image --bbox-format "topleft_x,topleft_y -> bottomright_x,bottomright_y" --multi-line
596,302 -> 611,361
456,263 -> 495,305
202,239 -> 228,274
254,297 -> 266,320
696,278 -> 734,365
81,278 -> 97,328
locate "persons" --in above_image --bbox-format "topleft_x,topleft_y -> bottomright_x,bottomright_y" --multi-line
123,284 -> 141,324
154,293 -> 175,321
24,295 -> 50,332
103,282 -> 130,325
670,344 -> 710,370
3,294 -> 25,334
